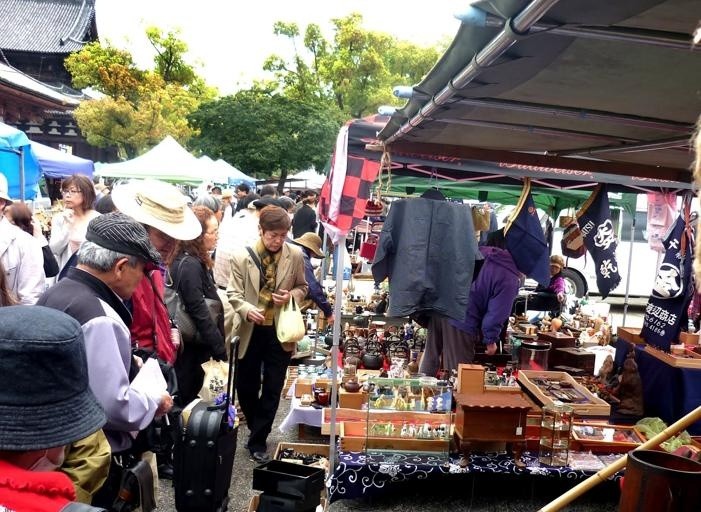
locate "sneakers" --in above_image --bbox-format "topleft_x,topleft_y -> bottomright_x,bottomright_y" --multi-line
158,464 -> 174,479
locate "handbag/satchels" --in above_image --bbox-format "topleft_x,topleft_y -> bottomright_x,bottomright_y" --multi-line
198,357 -> 234,402
112,461 -> 156,512
277,293 -> 305,343
164,287 -> 221,342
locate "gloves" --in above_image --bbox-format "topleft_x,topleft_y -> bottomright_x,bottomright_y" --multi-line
211,342 -> 228,362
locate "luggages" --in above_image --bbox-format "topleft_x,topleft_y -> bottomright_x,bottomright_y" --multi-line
173,336 -> 240,512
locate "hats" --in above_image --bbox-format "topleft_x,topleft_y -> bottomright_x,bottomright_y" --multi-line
0,304 -> 108,451
0,173 -> 13,205
253,198 -> 287,210
291,232 -> 325,259
86,210 -> 162,264
111,180 -> 202,240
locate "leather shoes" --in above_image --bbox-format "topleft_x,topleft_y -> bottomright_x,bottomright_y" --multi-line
244,430 -> 269,463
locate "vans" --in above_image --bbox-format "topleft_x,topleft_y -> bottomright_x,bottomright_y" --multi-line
494,197 -> 678,306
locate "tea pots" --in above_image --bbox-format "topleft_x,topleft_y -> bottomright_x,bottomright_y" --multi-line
340,326 -> 409,370
340,379 -> 364,393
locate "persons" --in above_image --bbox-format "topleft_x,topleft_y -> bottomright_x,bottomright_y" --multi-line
355,233 -> 379,274
0,171 -> 324,512
418,243 -> 528,378
533,254 -> 565,303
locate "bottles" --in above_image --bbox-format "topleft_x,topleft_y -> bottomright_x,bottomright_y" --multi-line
408,358 -> 418,372
407,326 -> 415,339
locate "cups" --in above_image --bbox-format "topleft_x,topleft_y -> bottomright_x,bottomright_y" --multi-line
315,387 -> 329,406
687,334 -> 699,345
302,393 -> 312,406
615,448 -> 701,511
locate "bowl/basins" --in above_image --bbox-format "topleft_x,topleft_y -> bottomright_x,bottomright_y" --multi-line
325,333 -> 343,345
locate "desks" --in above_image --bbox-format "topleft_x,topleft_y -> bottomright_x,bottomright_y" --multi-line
246,282 -> 699,512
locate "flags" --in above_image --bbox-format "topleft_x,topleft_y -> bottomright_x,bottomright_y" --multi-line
314,153 -> 381,247
646,193 -> 679,255
639,216 -> 693,351
503,177 -> 552,290
573,183 -> 622,300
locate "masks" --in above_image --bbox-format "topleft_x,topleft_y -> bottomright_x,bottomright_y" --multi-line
27,445 -> 66,471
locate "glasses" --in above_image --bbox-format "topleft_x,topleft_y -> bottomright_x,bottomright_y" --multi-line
60,189 -> 81,195
